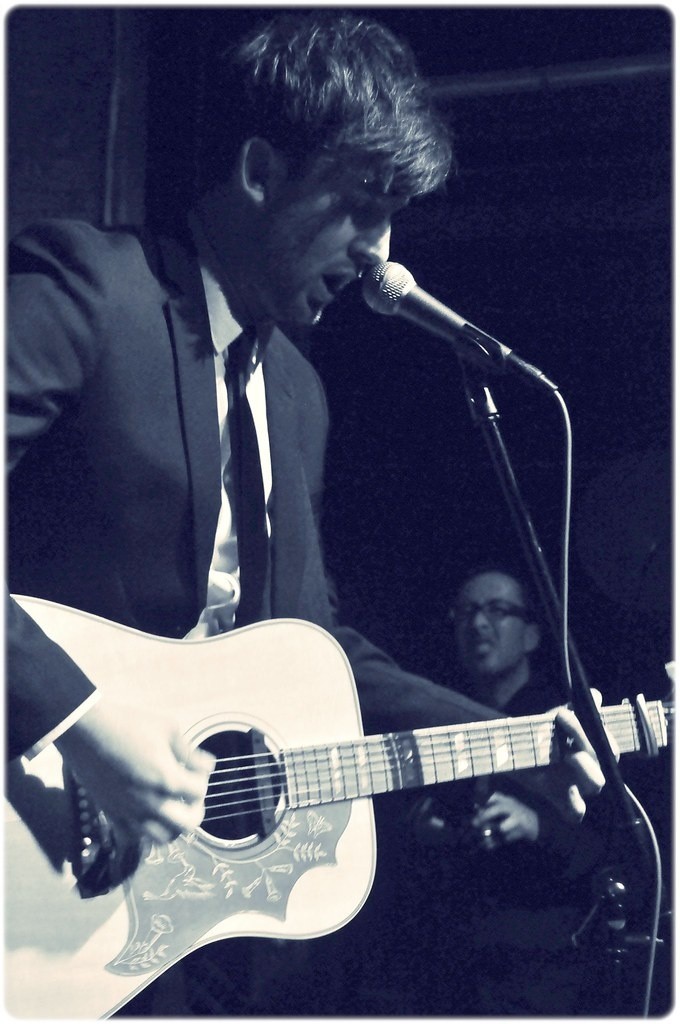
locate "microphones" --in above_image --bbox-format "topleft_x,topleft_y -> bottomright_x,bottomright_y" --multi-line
363,261 -> 559,397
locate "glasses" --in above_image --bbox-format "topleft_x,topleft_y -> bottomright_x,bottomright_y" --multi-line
449,601 -> 530,620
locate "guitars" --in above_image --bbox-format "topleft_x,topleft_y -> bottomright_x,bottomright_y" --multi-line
3,590 -> 675,1020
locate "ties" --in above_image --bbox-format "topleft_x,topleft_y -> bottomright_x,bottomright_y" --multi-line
228,322 -> 270,630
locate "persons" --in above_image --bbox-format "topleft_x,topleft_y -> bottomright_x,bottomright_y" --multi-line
393,564 -> 614,1017
6,16 -> 620,1018
